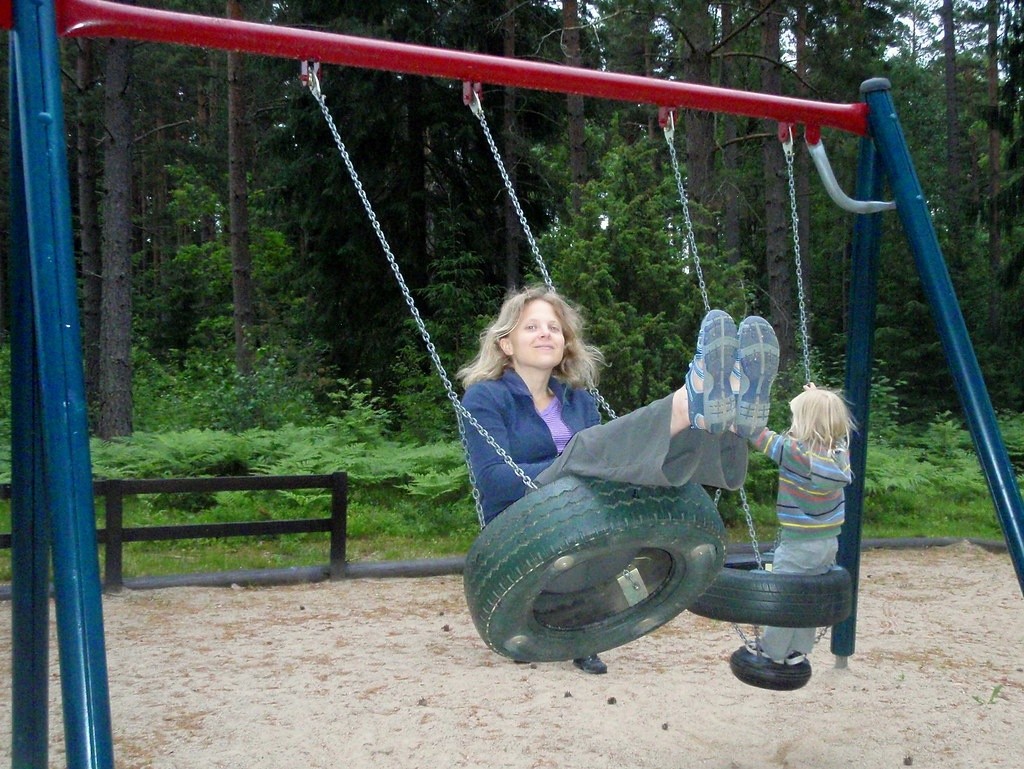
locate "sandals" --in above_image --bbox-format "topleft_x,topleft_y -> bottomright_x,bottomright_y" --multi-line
685,309 -> 780,438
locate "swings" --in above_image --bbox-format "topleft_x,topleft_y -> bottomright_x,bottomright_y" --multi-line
662,118 -> 857,688
298,81 -> 732,666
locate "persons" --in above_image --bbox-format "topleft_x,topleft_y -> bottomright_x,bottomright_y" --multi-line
744,383 -> 858,668
515,654 -> 607,675
456,288 -> 782,595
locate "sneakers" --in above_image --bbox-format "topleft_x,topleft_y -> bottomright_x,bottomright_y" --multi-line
746,643 -> 804,665
572,654 -> 607,673
